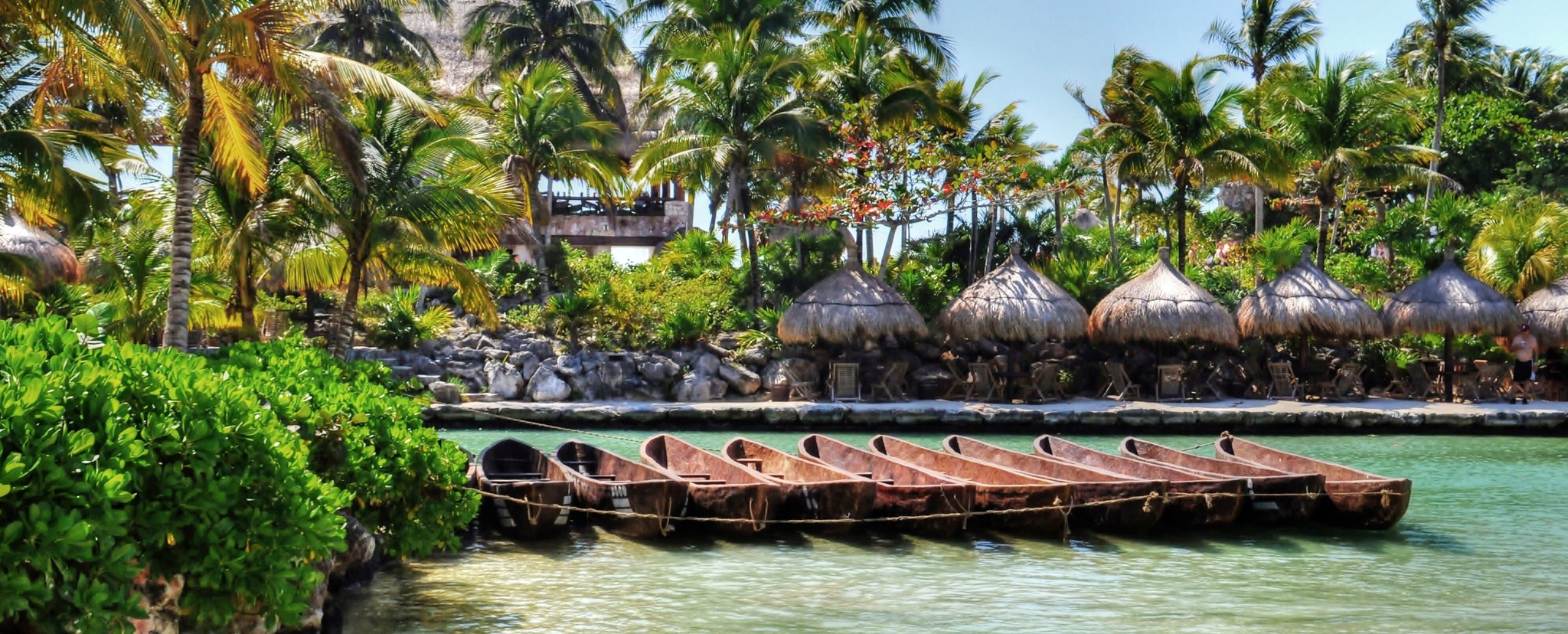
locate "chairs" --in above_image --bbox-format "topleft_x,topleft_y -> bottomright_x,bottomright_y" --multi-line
775,356 -> 1567,404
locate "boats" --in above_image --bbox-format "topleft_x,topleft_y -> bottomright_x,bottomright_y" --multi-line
1116,436 -> 1327,535
639,433 -> 781,542
397,434 -> 477,549
1030,431 -> 1249,542
551,435 -> 690,537
868,428 -> 1077,537
1213,431 -> 1413,544
475,437 -> 576,547
795,434 -> 978,540
720,435 -> 877,540
942,434 -> 1171,535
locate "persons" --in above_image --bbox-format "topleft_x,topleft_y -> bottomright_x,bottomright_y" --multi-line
1508,323 -> 1540,404
1545,348 -> 1568,384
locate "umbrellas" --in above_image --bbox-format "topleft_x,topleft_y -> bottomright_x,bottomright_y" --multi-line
1083,245 -> 1239,391
1372,256 -> 1523,401
1231,252 -> 1385,385
1513,275 -> 1568,379
775,255 -> 929,388
938,241 -> 1092,398
